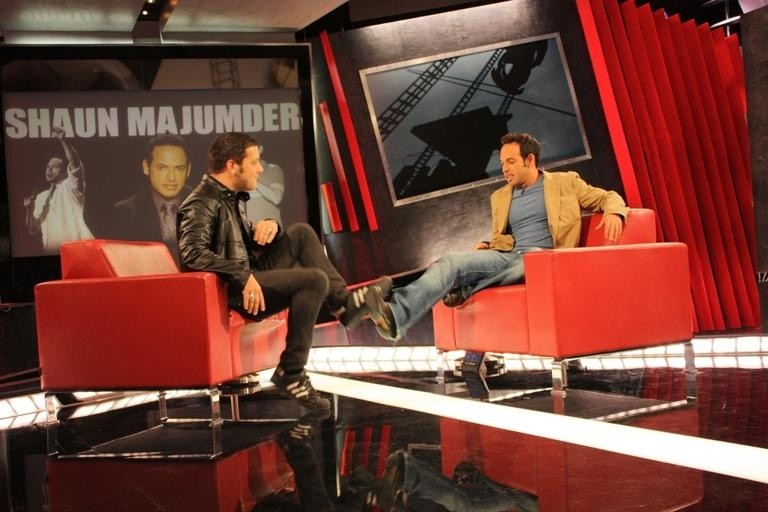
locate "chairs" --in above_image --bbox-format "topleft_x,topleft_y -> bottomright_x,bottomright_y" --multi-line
433,208 -> 698,392
438,374 -> 705,512
34,239 -> 297,463
44,425 -> 301,512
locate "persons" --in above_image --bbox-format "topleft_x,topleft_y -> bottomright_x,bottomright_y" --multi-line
23,127 -> 96,255
366,129 -> 629,343
237,134 -> 285,236
175,131 -> 393,410
248,410 -> 393,512
366,448 -> 539,512
113,135 -> 194,265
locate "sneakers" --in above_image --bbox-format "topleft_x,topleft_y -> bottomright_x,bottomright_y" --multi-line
274,411 -> 331,454
338,276 -> 394,336
443,286 -> 465,306
271,366 -> 330,409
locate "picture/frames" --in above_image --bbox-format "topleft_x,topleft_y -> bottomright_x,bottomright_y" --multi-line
359,30 -> 592,207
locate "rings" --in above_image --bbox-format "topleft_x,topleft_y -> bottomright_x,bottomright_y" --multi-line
268,228 -> 273,232
249,294 -> 255,299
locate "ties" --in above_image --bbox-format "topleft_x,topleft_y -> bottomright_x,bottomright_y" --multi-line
161,201 -> 181,272
